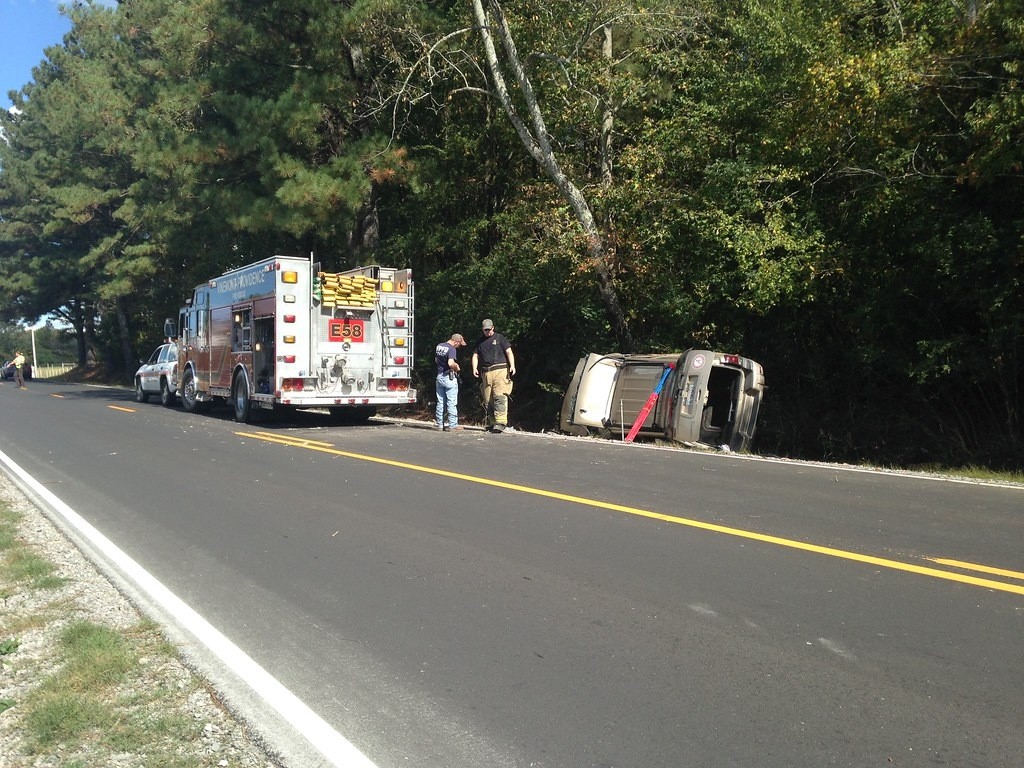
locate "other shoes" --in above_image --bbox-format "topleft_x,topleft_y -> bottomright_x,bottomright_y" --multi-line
448,426 -> 464,431
438,428 -> 445,431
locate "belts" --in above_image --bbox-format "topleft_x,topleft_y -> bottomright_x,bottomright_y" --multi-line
482,365 -> 509,372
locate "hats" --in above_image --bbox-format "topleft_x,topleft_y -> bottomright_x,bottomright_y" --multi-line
451,334 -> 467,346
482,319 -> 493,330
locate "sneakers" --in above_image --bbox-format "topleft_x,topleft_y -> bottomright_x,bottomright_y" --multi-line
492,424 -> 505,431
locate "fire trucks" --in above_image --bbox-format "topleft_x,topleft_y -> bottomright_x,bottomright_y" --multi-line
164,251 -> 419,426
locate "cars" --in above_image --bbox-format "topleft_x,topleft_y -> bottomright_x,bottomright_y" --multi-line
0,361 -> 32,381
559,346 -> 769,454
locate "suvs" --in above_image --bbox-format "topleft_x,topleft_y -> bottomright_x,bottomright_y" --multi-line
134,343 -> 179,409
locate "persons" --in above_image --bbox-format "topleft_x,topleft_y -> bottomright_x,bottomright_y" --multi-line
434,333 -> 467,431
7,351 -> 25,387
472,319 -> 515,432
